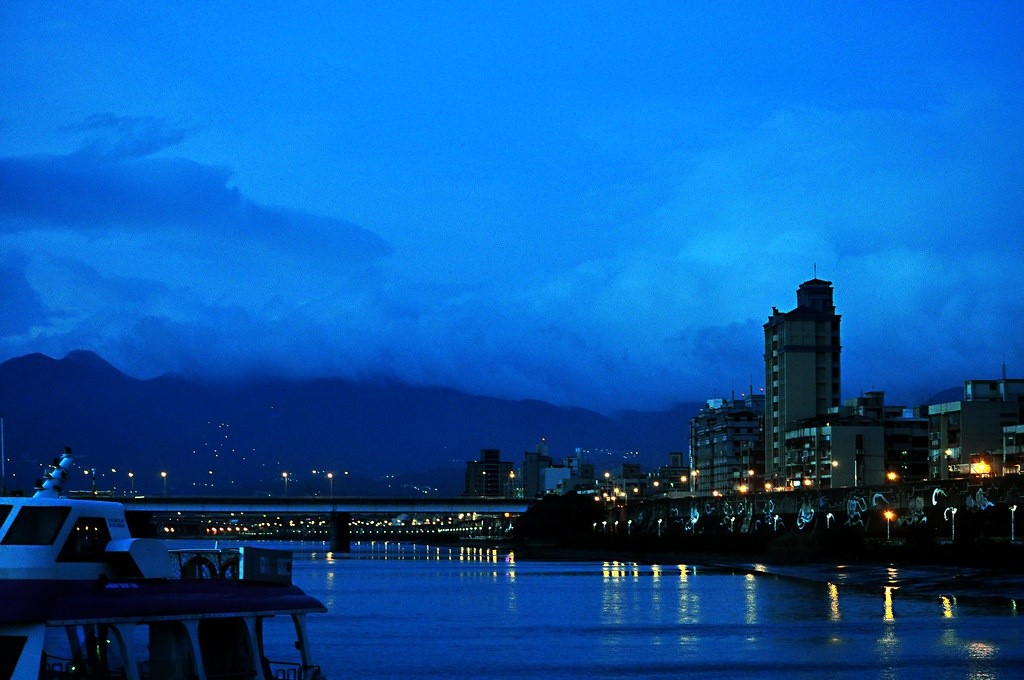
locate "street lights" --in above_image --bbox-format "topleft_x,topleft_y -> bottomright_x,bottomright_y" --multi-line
951,507 -> 958,540
160,471 -> 166,498
128,472 -> 133,493
602,521 -> 608,533
326,473 -> 333,496
593,522 -> 597,531
604,472 -> 610,495
614,521 -> 618,533
826,513 -> 832,528
1009,505 -> 1017,540
628,520 -> 632,535
885,511 -> 897,540
731,517 -> 735,533
282,472 -> 288,496
658,519 -> 662,536
774,515 -> 778,531
691,518 -> 696,534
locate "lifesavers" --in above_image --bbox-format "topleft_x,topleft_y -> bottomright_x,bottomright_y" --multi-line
181,556 -> 216,578
221,558 -> 241,582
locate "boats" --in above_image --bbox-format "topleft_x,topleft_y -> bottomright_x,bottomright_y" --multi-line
0,449 -> 328,680
